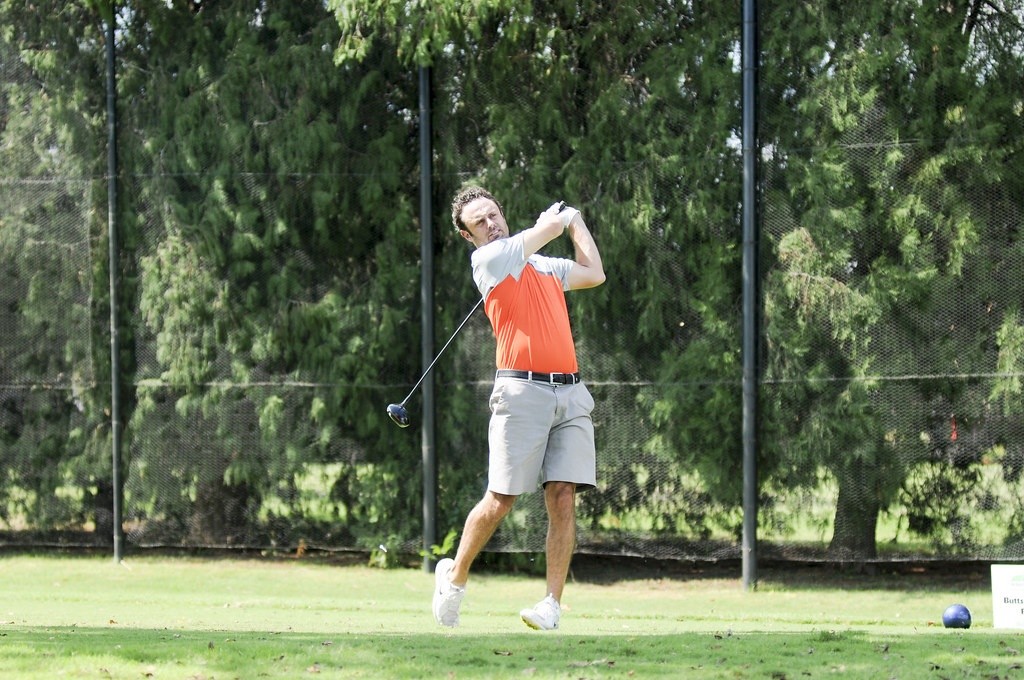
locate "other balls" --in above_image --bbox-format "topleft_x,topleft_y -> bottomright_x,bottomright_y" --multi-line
942,603 -> 971,629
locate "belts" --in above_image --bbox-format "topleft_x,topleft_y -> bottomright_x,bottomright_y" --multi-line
497,369 -> 580,384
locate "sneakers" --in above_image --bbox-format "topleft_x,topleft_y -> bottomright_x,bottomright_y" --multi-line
522,593 -> 561,630
433,558 -> 466,625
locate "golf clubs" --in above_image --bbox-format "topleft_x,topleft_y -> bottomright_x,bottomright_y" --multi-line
386,200 -> 568,428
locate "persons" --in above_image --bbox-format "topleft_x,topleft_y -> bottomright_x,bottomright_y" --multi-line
432,187 -> 606,628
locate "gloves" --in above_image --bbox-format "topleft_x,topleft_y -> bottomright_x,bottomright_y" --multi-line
547,202 -> 581,228
